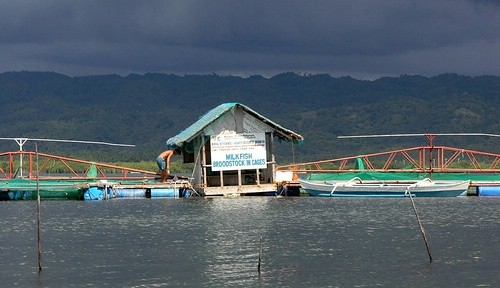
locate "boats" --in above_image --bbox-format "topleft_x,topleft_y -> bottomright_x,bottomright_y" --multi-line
298,178 -> 470,197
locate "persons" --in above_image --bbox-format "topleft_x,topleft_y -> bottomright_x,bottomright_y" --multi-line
156,148 -> 181,184
